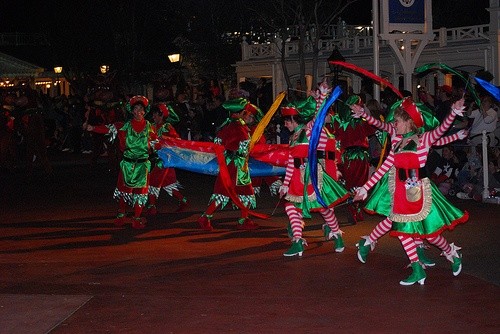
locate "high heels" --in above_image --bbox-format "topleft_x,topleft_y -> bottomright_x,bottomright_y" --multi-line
333,234 -> 346,253
115,216 -> 130,225
283,240 -> 305,257
323,224 -> 333,240
145,206 -> 158,215
451,249 -> 463,276
346,207 -> 368,224
237,217 -> 258,229
399,262 -> 427,286
176,200 -> 191,212
198,217 -> 215,233
130,219 -> 145,228
355,239 -> 371,264
416,247 -> 436,267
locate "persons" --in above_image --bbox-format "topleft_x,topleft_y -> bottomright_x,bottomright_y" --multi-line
279,94 -> 469,286
199,97 -> 267,230
1,70 -> 500,202
88,95 -> 191,229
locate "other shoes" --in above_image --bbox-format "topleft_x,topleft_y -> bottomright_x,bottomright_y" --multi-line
456,192 -> 472,200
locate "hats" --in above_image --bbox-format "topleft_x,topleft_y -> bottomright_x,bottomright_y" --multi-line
279,96 -> 317,119
158,103 -> 180,125
222,97 -> 256,114
126,95 -> 150,118
401,97 -> 440,129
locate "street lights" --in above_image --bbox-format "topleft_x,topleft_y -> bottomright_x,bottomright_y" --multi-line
53,64 -> 64,97
326,46 -> 346,115
167,47 -> 184,99
100,64 -> 110,90
